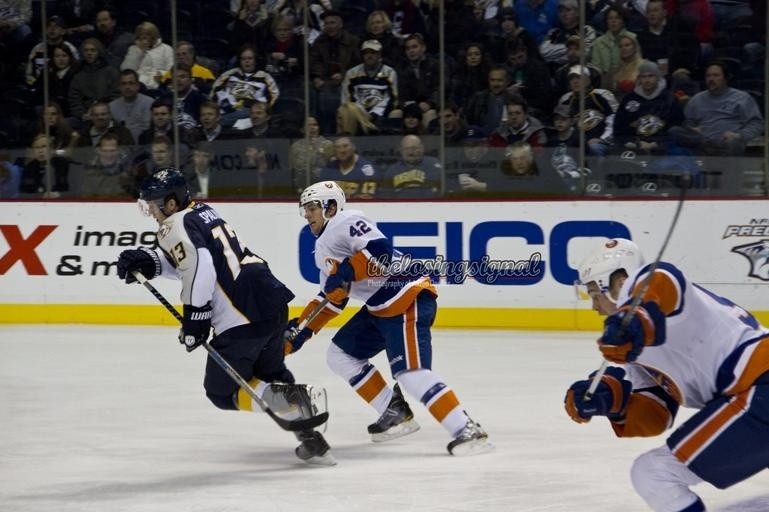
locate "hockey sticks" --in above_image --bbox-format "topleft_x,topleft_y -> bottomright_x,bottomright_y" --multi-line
580,171 -> 691,401
135,271 -> 330,430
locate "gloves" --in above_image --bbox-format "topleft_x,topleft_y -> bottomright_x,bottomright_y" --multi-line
323,256 -> 356,306
564,366 -> 633,424
116,246 -> 162,284
284,317 -> 314,356
596,297 -> 667,365
178,304 -> 213,352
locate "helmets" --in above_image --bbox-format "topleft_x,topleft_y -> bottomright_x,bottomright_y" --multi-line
577,237 -> 645,305
297,180 -> 346,222
137,168 -> 192,216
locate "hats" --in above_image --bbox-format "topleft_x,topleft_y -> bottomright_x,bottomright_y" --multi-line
46,0 -> 661,142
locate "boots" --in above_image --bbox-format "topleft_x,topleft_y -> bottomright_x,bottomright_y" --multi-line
270,381 -> 315,426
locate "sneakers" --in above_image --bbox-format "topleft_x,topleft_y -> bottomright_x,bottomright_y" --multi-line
367,381 -> 415,435
295,430 -> 331,461
446,420 -> 490,455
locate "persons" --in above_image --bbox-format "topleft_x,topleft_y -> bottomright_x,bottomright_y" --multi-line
282,177 -> 491,454
114,167 -> 334,464
561,237 -> 769,510
0,2 -> 769,201
